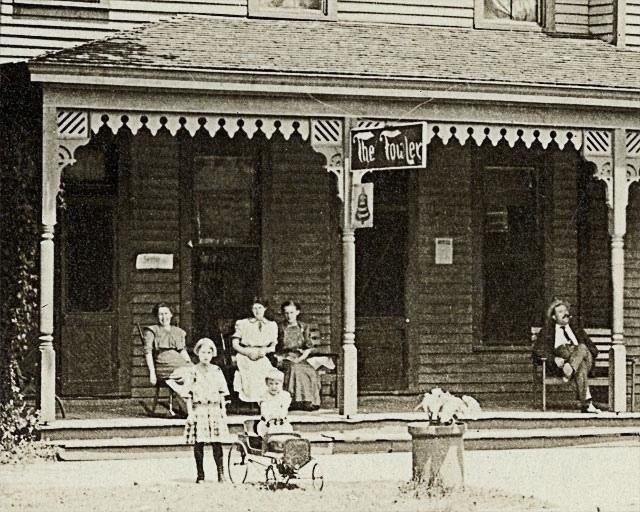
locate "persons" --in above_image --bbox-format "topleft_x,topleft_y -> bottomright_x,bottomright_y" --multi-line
141,302 -> 193,415
181,337 -> 233,485
232,299 -> 280,404
270,299 -> 323,412
255,372 -> 292,444
532,299 -> 601,414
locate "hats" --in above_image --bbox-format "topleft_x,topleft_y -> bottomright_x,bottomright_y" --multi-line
547,300 -> 570,317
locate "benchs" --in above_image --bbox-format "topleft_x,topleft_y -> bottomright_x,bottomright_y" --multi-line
530,325 -> 636,412
216,317 -> 341,415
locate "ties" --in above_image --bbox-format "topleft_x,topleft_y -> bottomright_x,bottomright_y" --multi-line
560,326 -> 573,345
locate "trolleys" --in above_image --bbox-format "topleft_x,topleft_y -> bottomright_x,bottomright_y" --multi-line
229,420 -> 325,491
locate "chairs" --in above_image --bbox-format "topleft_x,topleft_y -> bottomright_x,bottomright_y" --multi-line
135,318 -> 199,419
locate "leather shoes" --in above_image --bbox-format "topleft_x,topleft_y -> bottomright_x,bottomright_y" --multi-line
581,404 -> 601,413
563,363 -> 573,382
